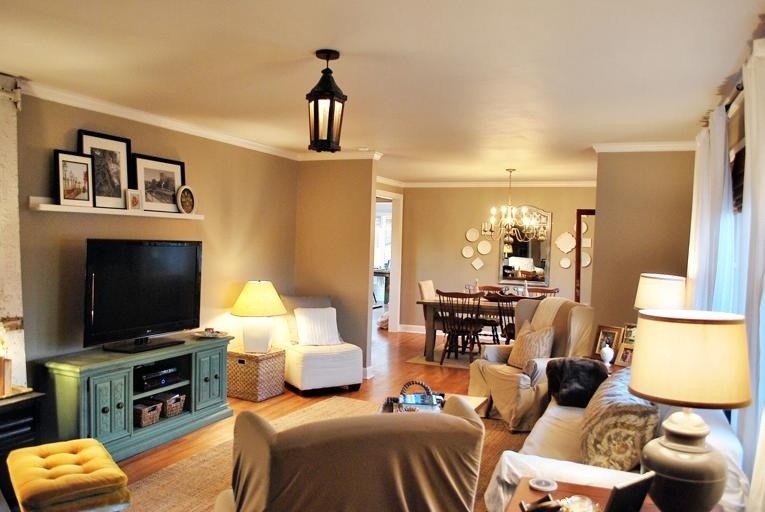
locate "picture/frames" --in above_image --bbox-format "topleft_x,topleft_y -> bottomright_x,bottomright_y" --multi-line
591,324 -> 624,364
603,469 -> 656,512
131,152 -> 186,213
53,149 -> 96,208
125,189 -> 142,211
613,343 -> 633,367
175,185 -> 197,214
77,129 -> 131,209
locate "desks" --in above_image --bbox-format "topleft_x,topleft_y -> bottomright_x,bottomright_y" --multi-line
415,291 -> 517,362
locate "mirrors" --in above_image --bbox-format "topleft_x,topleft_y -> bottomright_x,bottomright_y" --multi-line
499,205 -> 552,288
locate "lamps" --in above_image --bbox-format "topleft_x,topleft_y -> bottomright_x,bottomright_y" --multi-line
484,168 -> 540,249
628,308 -> 753,512
229,279 -> 288,354
633,272 -> 687,311
305,49 -> 348,153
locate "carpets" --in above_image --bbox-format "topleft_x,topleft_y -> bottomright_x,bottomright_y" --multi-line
125,394 -> 528,512
405,339 -> 483,369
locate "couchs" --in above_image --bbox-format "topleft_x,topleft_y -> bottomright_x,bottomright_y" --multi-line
214,394 -> 486,512
508,256 -> 533,270
484,361 -> 749,512
467,296 -> 594,431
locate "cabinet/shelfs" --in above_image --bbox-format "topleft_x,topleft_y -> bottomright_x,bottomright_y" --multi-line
131,352 -> 195,432
79,369 -> 132,445
191,343 -> 227,413
0,391 -> 47,480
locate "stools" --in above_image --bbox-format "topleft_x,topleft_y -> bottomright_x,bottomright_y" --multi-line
285,343 -> 363,397
5,438 -> 133,512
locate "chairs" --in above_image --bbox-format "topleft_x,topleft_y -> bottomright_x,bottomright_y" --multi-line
520,270 -> 532,277
473,286 -> 506,344
523,287 -> 559,298
482,295 -> 546,344
435,289 -> 485,365
418,280 -> 457,356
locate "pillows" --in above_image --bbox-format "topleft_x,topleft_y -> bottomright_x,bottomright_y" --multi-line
507,318 -> 554,368
279,294 -> 344,346
576,365 -> 661,474
293,306 -> 342,346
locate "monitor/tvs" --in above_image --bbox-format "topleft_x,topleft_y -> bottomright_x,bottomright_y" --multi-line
82,238 -> 202,354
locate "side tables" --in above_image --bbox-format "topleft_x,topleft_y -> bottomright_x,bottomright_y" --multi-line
502,476 -> 661,512
